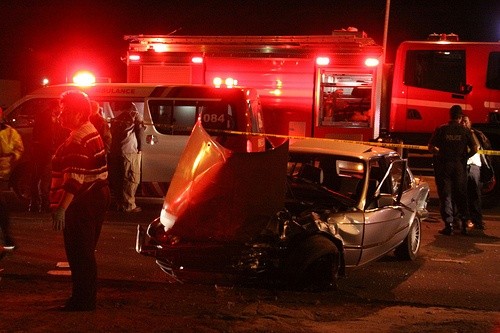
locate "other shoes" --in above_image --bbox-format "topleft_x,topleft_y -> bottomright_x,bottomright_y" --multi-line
461,226 -> 468,234
441,224 -> 454,234
119,207 -> 142,214
58,297 -> 96,310
5,239 -> 16,249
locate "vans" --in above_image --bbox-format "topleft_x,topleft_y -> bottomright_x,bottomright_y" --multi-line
0,82 -> 266,206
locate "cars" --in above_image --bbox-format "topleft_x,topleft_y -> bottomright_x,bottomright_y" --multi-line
135,117 -> 431,292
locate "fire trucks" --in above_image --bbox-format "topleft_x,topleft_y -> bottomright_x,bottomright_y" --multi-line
123,27 -> 500,209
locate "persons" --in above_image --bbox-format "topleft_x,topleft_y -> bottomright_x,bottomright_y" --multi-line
0,89 -> 144,310
428,106 -> 492,235
